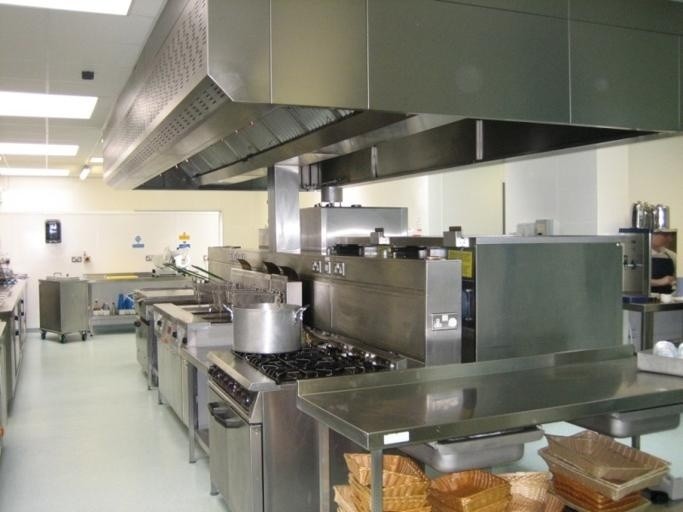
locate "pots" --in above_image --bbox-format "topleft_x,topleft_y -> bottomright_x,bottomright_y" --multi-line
220,302 -> 312,354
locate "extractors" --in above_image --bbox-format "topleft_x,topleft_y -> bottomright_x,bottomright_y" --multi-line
99,0 -> 682,194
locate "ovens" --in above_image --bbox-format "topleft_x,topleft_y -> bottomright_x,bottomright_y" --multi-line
204,386 -> 264,511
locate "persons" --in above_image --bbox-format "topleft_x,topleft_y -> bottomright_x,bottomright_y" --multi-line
651,232 -> 677,294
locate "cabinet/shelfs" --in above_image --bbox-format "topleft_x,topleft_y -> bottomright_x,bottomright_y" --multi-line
620,226 -> 676,300
84,274 -> 199,332
0,278 -> 27,398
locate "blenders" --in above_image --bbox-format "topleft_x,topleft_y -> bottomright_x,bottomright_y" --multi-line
145,244 -> 182,277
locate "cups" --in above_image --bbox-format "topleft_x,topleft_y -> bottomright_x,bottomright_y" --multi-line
674,277 -> 682,296
650,489 -> 667,503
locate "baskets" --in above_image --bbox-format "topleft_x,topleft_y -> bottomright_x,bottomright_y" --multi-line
333,428 -> 673,512
331,428 -> 673,512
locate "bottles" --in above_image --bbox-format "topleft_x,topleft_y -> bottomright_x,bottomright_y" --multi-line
356,244 -> 446,260
117,293 -> 133,309
93,299 -> 117,316
464,286 -> 474,322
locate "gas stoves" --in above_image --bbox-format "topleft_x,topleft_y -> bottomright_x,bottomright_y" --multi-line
205,326 -> 404,425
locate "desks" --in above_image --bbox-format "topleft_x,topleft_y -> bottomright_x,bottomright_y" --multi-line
291,341 -> 681,511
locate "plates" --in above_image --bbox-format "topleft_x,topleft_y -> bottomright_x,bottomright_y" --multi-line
652,340 -> 683,359
